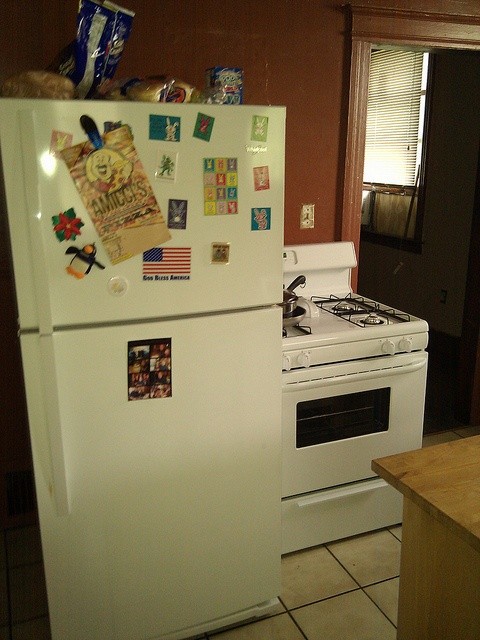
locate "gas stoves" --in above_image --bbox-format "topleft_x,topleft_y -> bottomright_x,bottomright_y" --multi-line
282,272 -> 430,372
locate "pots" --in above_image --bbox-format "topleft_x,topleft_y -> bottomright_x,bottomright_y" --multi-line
283,275 -> 306,314
283,306 -> 306,328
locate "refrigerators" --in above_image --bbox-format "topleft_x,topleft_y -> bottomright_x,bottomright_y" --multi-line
1,98 -> 284,640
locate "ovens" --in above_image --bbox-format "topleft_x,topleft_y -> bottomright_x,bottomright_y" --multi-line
281,350 -> 429,555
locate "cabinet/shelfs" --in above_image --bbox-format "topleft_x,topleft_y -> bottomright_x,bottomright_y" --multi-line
370,433 -> 480,640
